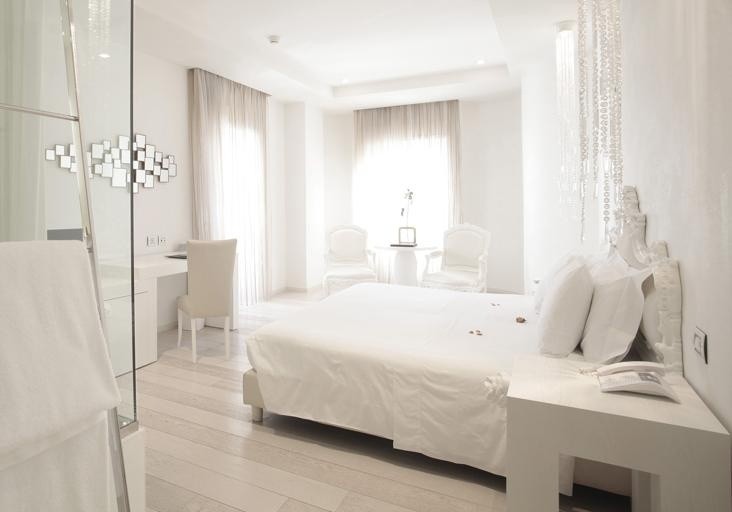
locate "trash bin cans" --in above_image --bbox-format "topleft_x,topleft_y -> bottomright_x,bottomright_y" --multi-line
177,312 -> 205,331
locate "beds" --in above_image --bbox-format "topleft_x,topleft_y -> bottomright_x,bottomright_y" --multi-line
242,186 -> 680,499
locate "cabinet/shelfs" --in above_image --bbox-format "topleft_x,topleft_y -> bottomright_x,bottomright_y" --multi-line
101,277 -> 159,378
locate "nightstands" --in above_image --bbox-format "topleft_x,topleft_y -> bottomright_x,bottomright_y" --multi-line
506,365 -> 731,511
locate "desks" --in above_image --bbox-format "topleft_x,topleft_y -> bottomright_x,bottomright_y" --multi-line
99,251 -> 239,370
380,246 -> 436,288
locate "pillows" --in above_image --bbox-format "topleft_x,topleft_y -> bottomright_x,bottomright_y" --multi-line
532,248 -> 592,360
584,248 -> 655,373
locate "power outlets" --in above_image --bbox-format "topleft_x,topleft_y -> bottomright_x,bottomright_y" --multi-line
147,235 -> 158,247
693,327 -> 706,362
158,235 -> 168,248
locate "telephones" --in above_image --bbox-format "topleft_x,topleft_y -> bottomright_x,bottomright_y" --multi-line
597,361 -> 677,401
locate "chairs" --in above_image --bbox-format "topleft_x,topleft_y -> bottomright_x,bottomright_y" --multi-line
176,237 -> 238,364
425,224 -> 493,293
324,224 -> 377,291
